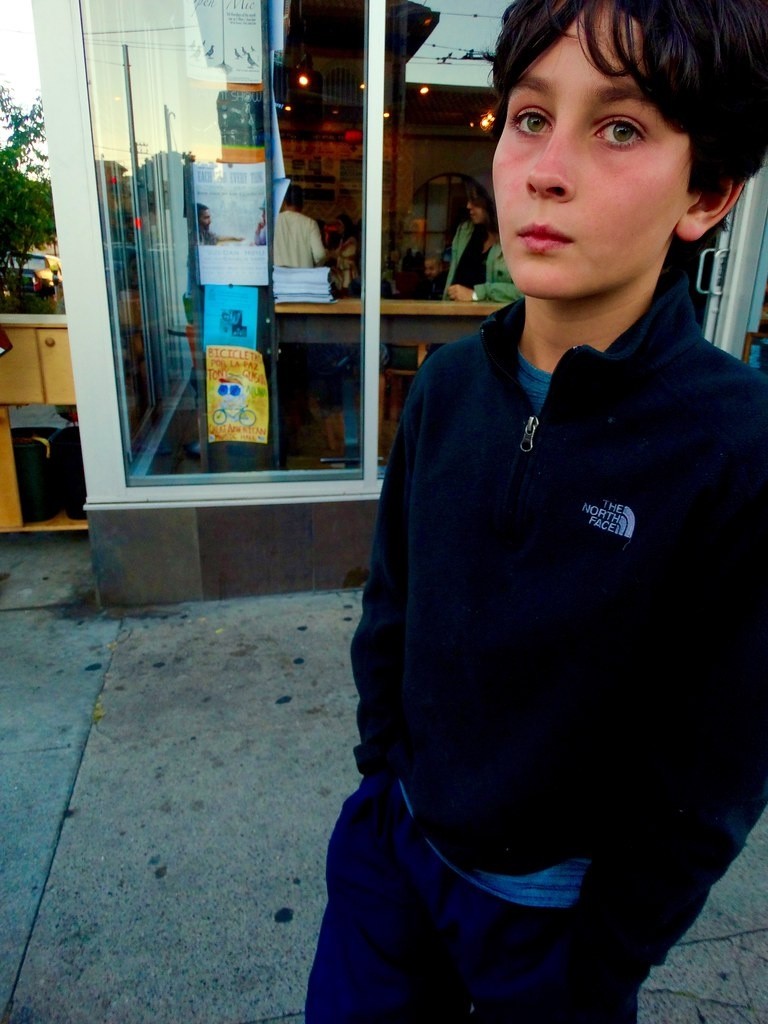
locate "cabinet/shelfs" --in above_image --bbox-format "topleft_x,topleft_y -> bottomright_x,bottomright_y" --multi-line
0,314 -> 88,533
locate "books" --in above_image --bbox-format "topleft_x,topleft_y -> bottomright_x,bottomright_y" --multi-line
183,1 -> 270,449
272,265 -> 338,304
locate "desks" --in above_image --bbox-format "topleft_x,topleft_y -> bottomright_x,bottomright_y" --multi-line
275,299 -> 520,468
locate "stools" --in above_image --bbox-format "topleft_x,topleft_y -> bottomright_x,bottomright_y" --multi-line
378,368 -> 417,439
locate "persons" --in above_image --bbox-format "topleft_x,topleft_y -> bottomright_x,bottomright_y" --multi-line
275,170 -> 327,266
308,0 -> 768,1024
328,211 -> 449,301
254,199 -> 266,246
420,176 -> 524,366
197,203 -> 245,245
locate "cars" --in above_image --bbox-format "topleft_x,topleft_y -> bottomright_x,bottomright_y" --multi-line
104,241 -> 168,289
0,252 -> 63,298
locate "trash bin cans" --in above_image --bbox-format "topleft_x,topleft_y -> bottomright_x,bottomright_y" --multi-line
11,427 -> 61,523
53,426 -> 89,520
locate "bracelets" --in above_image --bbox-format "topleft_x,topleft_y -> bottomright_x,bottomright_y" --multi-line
472,291 -> 478,301
